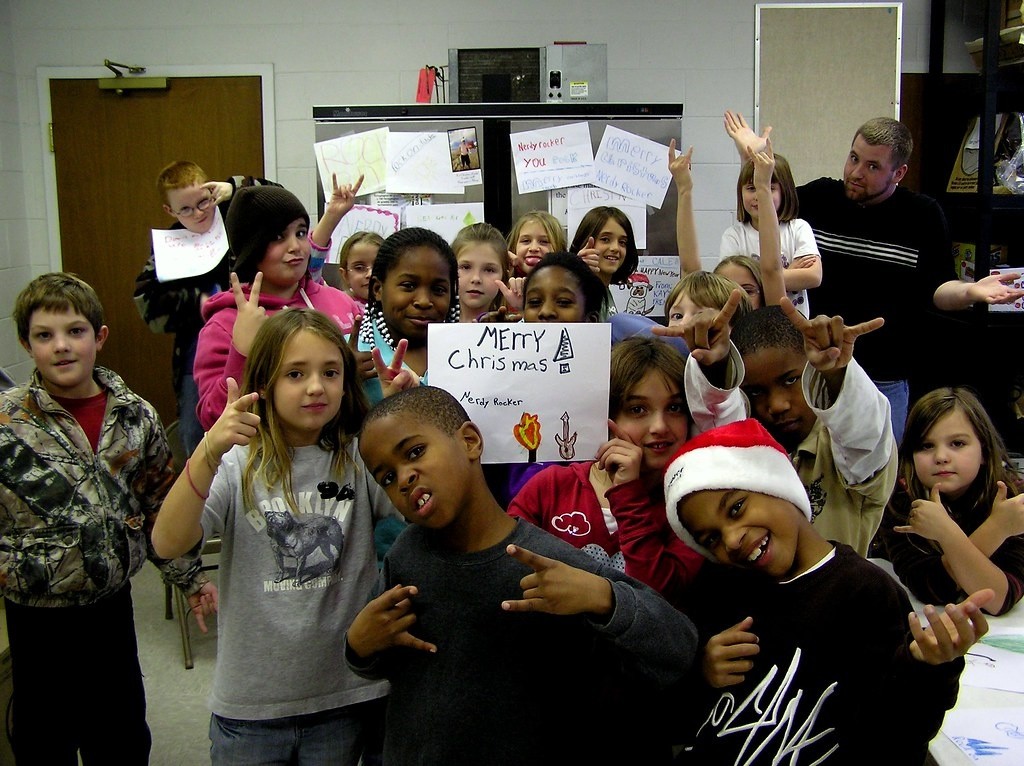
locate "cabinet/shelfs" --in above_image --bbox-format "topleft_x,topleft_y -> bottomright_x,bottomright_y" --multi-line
920,0 -> 1024,459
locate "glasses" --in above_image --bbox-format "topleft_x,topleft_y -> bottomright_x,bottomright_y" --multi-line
170,197 -> 212,216
743,286 -> 759,297
347,264 -> 373,274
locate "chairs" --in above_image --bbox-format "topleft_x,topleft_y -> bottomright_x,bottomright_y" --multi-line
164,420 -> 221,670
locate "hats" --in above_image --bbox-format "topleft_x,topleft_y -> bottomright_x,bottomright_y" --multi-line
663,418 -> 811,566
226,186 -> 310,272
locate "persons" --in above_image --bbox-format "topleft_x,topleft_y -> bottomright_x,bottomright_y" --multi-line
870,387 -> 1024,616
150,307 -> 420,766
343,386 -> 710,766
459,137 -> 473,169
0,272 -> 219,766
135,117 -> 1024,608
663,418 -> 997,766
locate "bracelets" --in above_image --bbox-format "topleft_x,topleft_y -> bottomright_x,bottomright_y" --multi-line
205,444 -> 219,475
182,458 -> 210,500
203,430 -> 222,465
